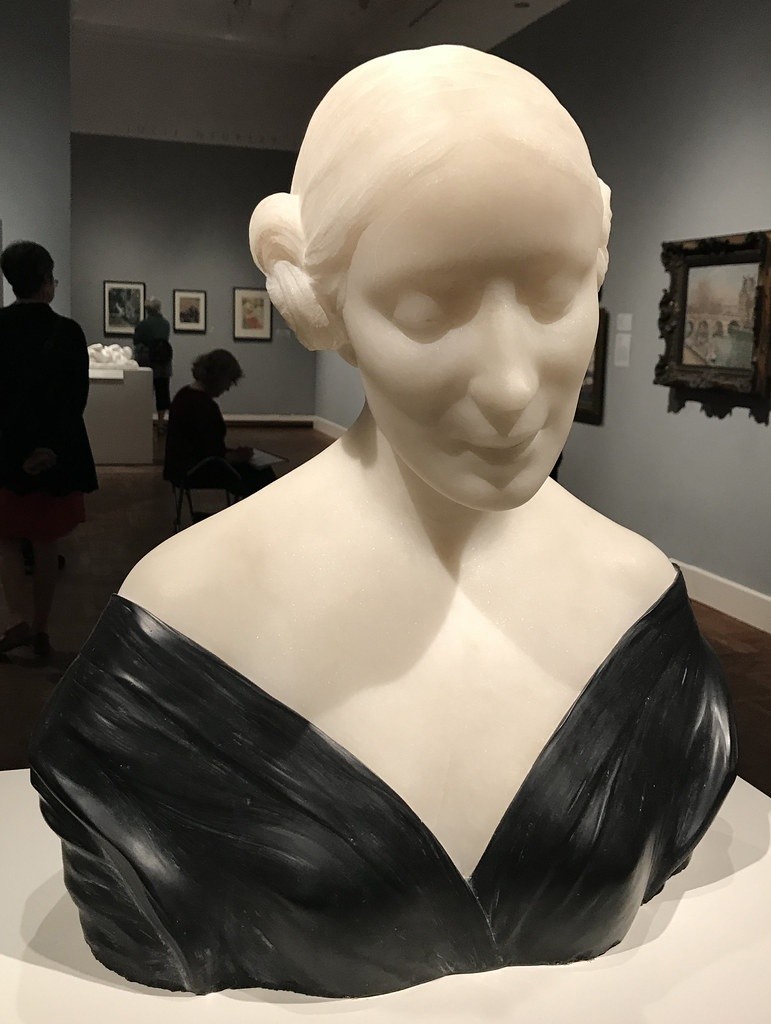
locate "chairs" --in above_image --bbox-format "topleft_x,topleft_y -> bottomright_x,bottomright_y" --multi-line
158,419 -> 240,534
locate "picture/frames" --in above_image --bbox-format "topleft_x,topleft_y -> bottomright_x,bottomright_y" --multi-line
652,230 -> 771,426
232,286 -> 273,343
173,289 -> 206,334
102,280 -> 146,335
574,308 -> 609,424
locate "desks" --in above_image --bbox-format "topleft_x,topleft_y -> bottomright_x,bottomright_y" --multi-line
0,767 -> 770,1023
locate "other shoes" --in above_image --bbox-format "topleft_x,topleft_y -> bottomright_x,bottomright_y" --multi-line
31,631 -> 50,655
0,622 -> 31,651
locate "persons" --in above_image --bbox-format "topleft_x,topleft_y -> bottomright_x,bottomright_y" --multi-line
0,242 -> 99,659
164,350 -> 276,496
29,44 -> 740,1000
134,298 -> 173,425
